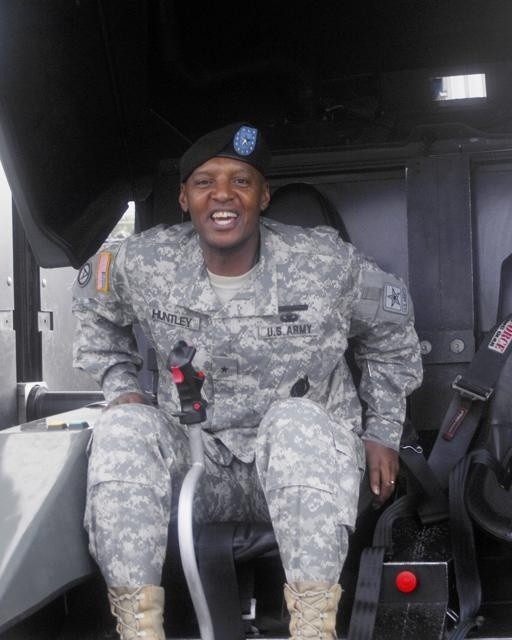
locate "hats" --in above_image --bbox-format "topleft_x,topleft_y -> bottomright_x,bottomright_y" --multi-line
178,121 -> 272,185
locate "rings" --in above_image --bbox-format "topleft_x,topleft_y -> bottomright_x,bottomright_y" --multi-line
389,480 -> 396,485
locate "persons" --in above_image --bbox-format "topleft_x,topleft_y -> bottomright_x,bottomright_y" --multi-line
69,123 -> 422,640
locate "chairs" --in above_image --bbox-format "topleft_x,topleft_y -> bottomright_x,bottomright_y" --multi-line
449,251 -> 510,553
167,181 -> 411,598
